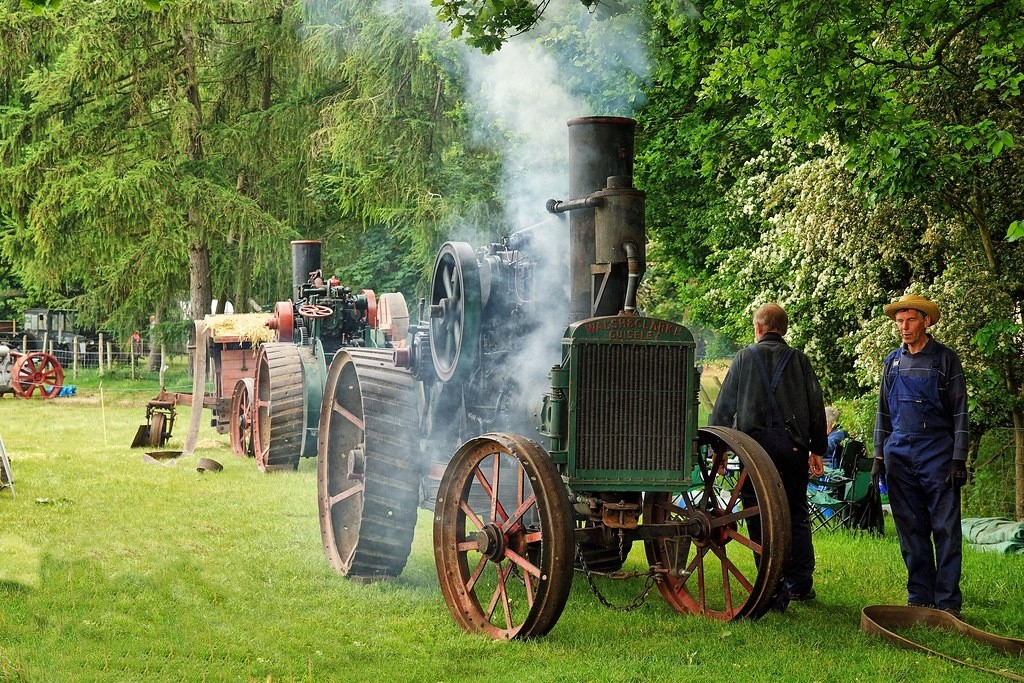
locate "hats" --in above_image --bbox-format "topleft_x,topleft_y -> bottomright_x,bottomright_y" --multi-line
883,296 -> 939,327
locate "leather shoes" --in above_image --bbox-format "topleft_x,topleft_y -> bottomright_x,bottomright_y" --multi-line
785,589 -> 815,600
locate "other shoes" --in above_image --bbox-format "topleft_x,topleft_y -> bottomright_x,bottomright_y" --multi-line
164,365 -> 170,373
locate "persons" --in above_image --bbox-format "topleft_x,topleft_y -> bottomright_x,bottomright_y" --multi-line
710,301 -> 829,613
871,294 -> 970,622
825,406 -> 849,472
150,316 -> 155,343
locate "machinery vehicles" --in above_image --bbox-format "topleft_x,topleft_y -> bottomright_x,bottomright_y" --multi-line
312,118 -> 791,646
21,309 -> 101,366
130,241 -> 413,474
0,337 -> 65,400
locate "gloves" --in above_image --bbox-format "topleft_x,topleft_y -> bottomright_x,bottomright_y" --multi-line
871,459 -> 886,493
946,460 -> 967,490
